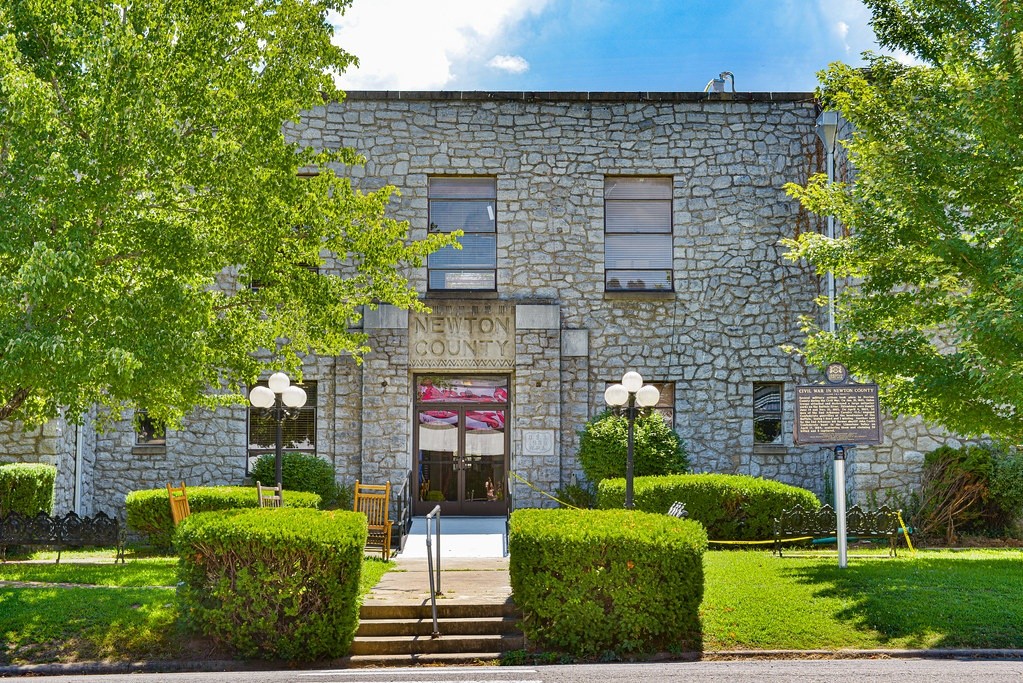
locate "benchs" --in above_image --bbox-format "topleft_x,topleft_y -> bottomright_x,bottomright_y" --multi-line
0,509 -> 127,565
772,504 -> 903,558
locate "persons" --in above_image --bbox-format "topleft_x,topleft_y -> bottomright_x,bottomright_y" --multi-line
485,477 -> 496,501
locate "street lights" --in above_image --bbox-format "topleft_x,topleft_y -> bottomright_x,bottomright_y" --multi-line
604,371 -> 661,509
250,371 -> 308,502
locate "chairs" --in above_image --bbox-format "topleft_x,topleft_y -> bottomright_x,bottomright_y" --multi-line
166,480 -> 190,527
353,479 -> 394,562
257,480 -> 283,507
